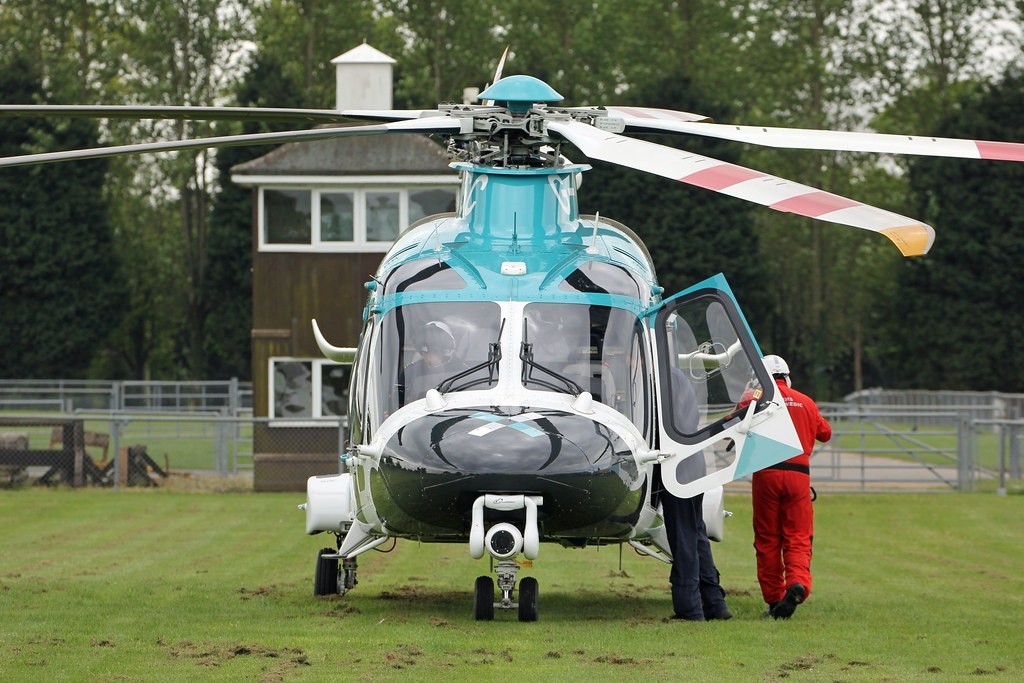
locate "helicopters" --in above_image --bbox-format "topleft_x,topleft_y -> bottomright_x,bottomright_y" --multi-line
1,40 -> 1024,623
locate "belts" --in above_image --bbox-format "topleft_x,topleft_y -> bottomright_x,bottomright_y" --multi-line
763,462 -> 813,474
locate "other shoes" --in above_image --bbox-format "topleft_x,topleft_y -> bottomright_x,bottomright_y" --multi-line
770,581 -> 807,621
667,607 -> 703,623
704,608 -> 733,622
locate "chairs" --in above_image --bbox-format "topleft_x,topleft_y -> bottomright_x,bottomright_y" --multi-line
562,363 -> 615,408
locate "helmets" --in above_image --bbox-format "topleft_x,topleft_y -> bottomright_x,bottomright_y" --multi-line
414,319 -> 457,360
750,356 -> 796,378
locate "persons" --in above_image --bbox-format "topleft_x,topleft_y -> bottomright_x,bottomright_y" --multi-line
625,343 -> 733,621
395,321 -> 476,410
731,355 -> 832,621
